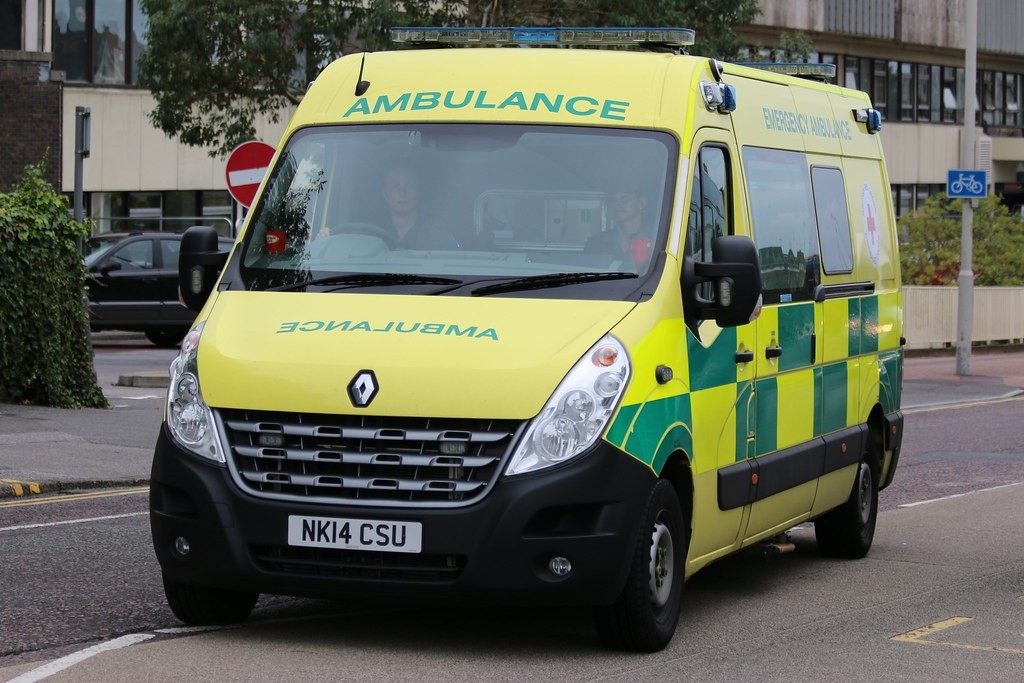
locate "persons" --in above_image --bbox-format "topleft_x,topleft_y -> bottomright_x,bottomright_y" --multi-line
316,159 -> 458,251
583,189 -> 653,260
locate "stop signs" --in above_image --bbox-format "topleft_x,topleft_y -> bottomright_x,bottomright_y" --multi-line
222,140 -> 279,210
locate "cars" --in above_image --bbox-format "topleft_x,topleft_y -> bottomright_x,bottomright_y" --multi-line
83,234 -> 238,347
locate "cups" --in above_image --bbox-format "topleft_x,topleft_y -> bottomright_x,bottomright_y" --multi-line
630,239 -> 648,261
266,229 -> 287,253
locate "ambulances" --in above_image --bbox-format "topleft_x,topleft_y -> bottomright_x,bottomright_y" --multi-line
149,41 -> 905,655
